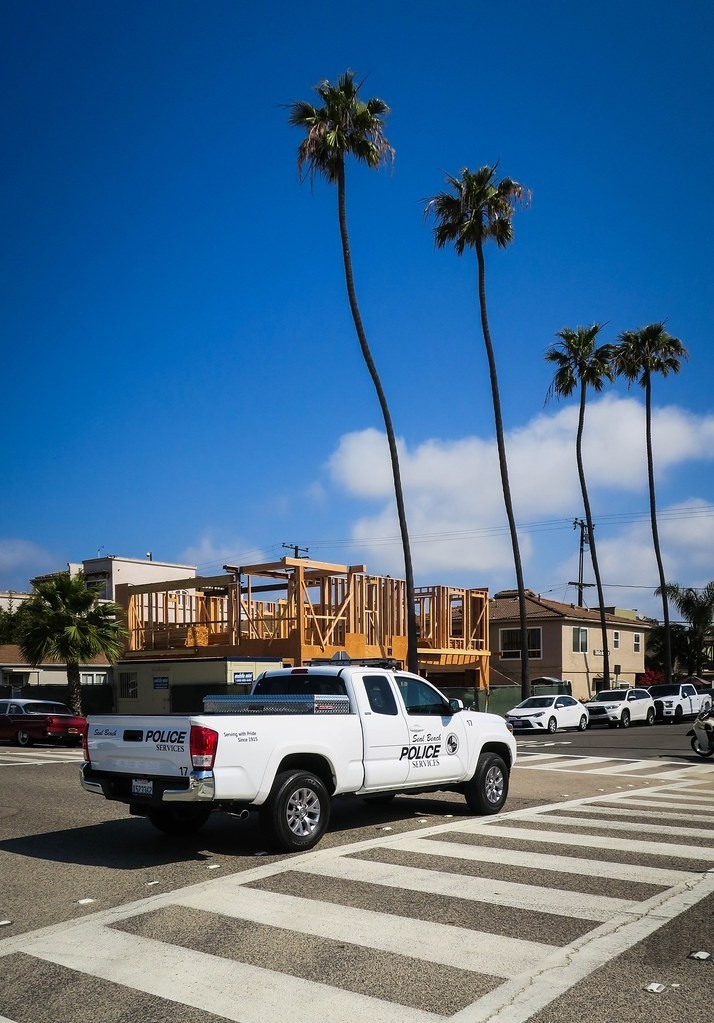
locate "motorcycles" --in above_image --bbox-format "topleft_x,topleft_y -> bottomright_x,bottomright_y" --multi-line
685,693 -> 714,757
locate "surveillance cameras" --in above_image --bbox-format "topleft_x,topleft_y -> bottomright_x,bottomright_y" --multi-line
146,553 -> 150,557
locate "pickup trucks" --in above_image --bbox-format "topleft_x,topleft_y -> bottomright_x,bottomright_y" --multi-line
75,666 -> 518,853
643,683 -> 712,725
0,697 -> 87,748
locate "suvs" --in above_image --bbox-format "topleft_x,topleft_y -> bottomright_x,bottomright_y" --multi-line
582,686 -> 657,730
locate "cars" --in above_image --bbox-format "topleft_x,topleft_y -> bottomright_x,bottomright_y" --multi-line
691,687 -> 714,718
503,694 -> 590,735
